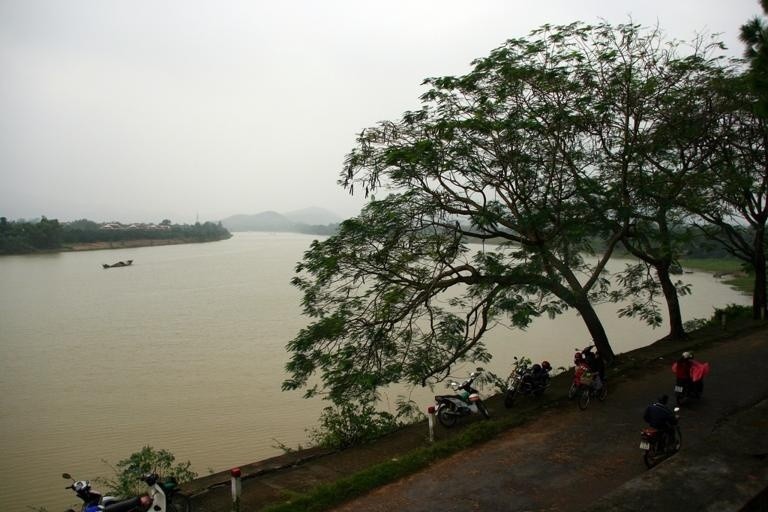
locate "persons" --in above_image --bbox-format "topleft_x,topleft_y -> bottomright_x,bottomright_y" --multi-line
588,351 -> 607,398
672,351 -> 710,393
643,393 -> 680,454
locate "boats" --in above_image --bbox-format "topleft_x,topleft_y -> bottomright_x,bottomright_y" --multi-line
103,260 -> 132,268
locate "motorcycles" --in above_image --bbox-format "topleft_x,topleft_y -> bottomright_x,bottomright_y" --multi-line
62,472 -> 120,512
435,367 -> 492,427
569,338 -> 608,410
639,399 -> 683,469
502,355 -> 551,409
672,351 -> 708,407
97,464 -> 194,512
